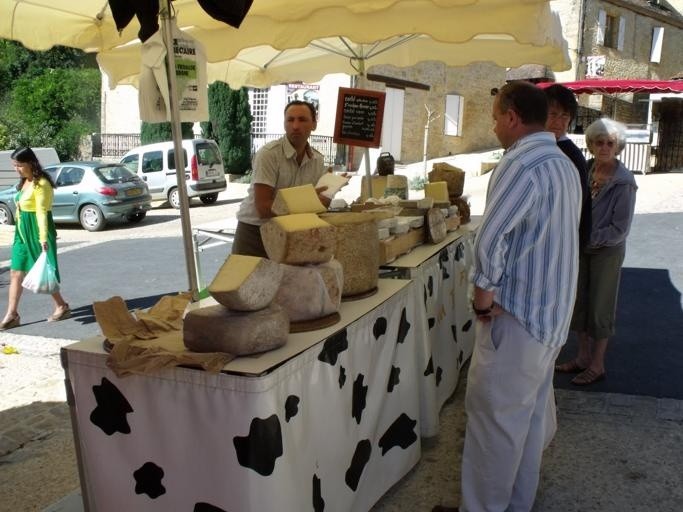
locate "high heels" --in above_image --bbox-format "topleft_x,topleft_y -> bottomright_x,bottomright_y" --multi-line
47,304 -> 71,322
0,313 -> 20,330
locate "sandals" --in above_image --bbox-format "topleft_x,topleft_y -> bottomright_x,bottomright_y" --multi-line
570,367 -> 607,384
554,358 -> 587,371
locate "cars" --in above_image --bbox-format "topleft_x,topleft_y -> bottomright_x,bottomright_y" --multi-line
0,162 -> 152,232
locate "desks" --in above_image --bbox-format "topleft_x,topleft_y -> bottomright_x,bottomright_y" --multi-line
61,214 -> 479,511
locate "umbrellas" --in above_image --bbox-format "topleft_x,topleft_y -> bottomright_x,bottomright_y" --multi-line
96,9 -> 571,198
1,0 -> 553,302
535,80 -> 683,120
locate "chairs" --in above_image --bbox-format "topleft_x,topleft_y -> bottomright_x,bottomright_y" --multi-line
58,171 -> 73,185
142,161 -> 153,173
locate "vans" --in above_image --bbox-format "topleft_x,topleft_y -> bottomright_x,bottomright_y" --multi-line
105,138 -> 226,209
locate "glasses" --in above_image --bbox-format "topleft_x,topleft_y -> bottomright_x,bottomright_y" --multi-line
591,139 -> 617,148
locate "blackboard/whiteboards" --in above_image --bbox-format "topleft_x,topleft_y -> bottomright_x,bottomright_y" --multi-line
333,87 -> 386,148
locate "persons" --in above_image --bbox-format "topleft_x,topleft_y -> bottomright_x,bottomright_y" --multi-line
433,80 -> 583,512
0,147 -> 71,331
231,101 -> 352,260
543,84 -> 593,256
555,118 -> 638,385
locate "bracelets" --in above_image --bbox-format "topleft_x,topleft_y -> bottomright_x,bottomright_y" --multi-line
473,301 -> 495,315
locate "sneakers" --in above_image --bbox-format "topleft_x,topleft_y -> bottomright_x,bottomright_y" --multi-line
431,505 -> 460,511
